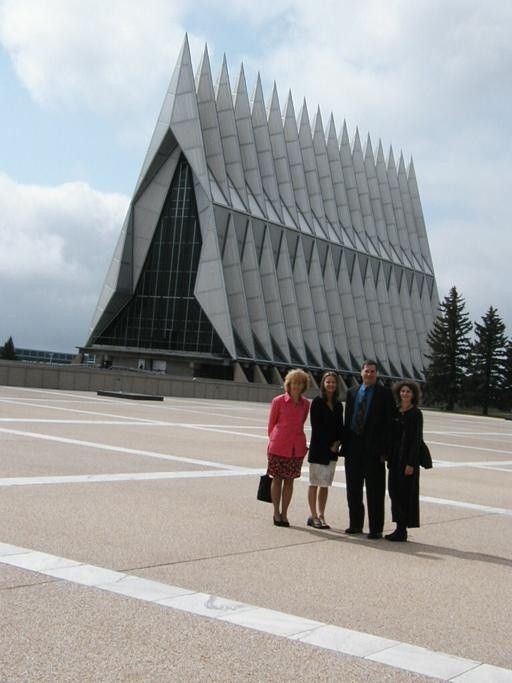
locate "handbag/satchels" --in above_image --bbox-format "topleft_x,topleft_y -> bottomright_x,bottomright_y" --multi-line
419,437 -> 434,469
256,469 -> 275,503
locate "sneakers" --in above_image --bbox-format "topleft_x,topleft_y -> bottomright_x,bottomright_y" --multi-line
345,524 -> 407,542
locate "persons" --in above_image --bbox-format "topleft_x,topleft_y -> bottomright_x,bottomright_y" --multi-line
379,381 -> 433,541
337,360 -> 387,539
265,367 -> 310,528
307,371 -> 344,530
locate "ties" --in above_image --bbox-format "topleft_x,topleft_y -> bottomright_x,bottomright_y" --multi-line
354,387 -> 369,427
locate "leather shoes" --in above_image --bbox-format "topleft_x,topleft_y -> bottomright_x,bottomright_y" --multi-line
273,513 -> 330,528
